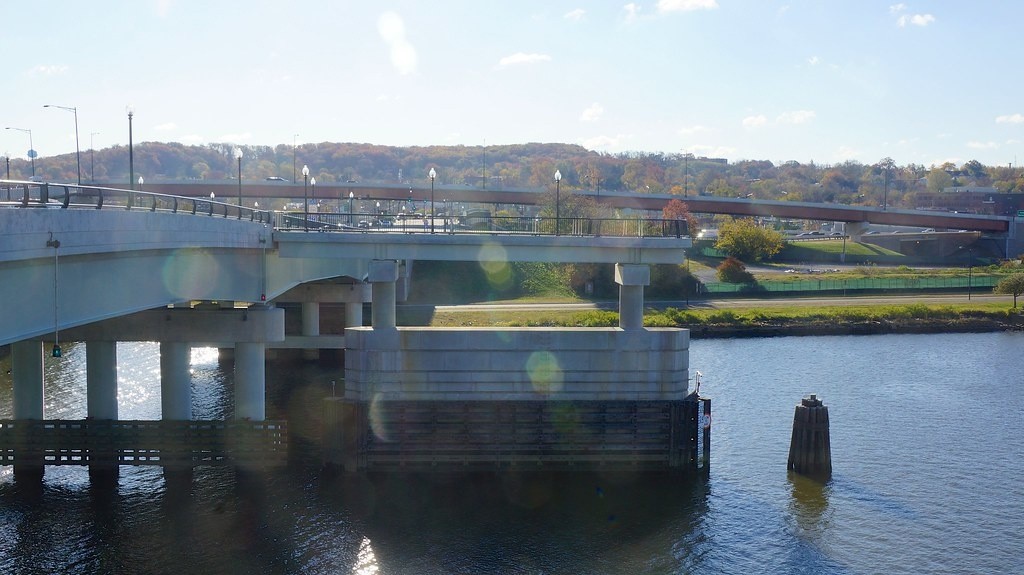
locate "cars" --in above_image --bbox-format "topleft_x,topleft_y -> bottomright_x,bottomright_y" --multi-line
264,176 -> 288,182
382,220 -> 392,228
783,267 -> 840,274
346,179 -> 359,184
357,220 -> 369,229
397,212 -> 433,220
795,227 -> 969,236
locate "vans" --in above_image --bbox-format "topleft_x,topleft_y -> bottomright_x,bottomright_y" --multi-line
696,229 -> 720,239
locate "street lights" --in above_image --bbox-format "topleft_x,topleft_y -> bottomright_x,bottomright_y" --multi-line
90,132 -> 101,182
255,202 -> 259,220
302,165 -> 315,231
210,191 -> 215,213
555,170 -> 565,236
43,104 -> 81,184
137,176 -> 144,206
235,148 -> 244,220
427,167 -> 438,234
402,205 -> 406,232
5,126 -> 35,178
127,108 -> 135,206
376,202 -> 381,231
350,191 -> 354,227
